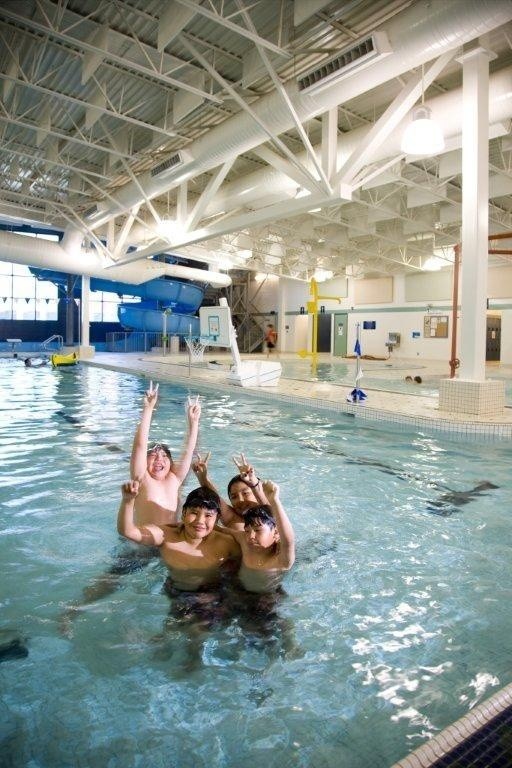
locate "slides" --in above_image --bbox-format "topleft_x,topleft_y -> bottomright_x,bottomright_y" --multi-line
1,246 -> 205,352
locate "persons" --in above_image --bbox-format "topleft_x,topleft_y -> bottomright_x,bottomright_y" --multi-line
24,358 -> 46,369
264,324 -> 279,359
405,376 -> 412,384
413,375 -> 422,384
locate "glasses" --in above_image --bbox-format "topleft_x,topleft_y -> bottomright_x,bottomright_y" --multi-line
246,508 -> 274,522
188,499 -> 219,509
147,442 -> 168,451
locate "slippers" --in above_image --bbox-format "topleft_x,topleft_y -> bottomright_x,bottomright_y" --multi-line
347,389 -> 368,403
